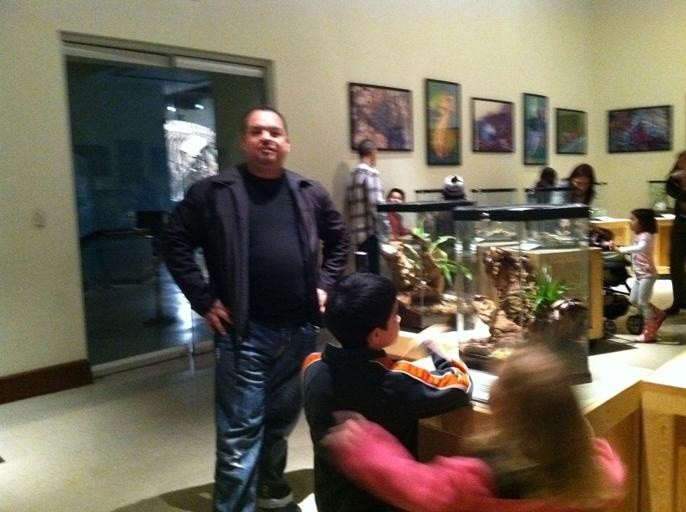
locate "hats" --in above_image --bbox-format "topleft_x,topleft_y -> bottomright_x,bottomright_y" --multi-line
443,174 -> 465,196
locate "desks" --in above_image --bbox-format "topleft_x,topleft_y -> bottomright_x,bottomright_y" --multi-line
393,328 -> 641,509
642,353 -> 686,510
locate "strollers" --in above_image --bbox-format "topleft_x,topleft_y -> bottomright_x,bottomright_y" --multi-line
589,224 -> 642,338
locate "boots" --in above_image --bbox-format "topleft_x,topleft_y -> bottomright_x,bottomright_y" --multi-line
650,303 -> 667,331
634,320 -> 657,344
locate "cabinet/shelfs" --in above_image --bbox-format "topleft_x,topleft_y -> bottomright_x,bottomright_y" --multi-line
454,202 -> 595,337
377,195 -> 478,338
594,215 -> 676,276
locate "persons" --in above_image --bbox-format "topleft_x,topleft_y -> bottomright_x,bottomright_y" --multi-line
161,106 -> 351,512
385,189 -> 412,240
435,174 -> 477,237
664,152 -> 686,316
568,164 -> 596,234
319,343 -> 629,512
618,208 -> 667,343
301,272 -> 474,512
527,167 -> 565,231
344,138 -> 392,275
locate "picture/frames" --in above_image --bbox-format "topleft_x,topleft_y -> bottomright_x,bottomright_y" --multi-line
522,93 -> 551,169
469,97 -> 519,156
554,107 -> 591,159
347,84 -> 418,152
426,80 -> 465,170
604,106 -> 674,154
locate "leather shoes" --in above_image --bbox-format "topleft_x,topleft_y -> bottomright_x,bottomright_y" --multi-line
664,306 -> 680,314
